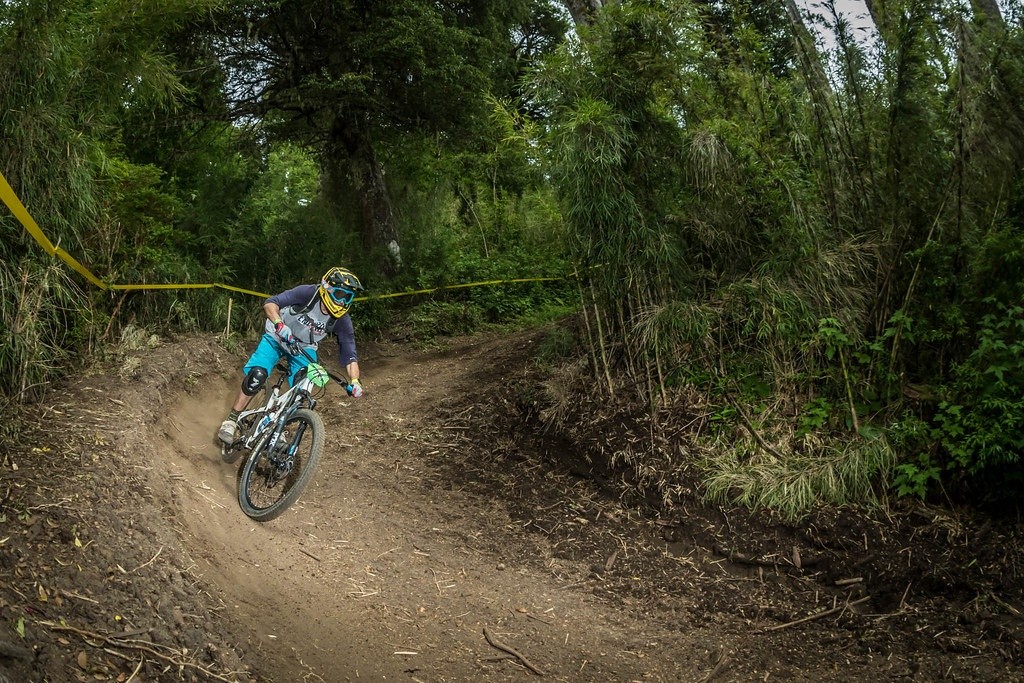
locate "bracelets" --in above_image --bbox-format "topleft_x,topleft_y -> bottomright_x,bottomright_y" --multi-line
273,320 -> 281,324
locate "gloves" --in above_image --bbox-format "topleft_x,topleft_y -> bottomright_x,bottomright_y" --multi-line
347,377 -> 363,398
273,319 -> 294,344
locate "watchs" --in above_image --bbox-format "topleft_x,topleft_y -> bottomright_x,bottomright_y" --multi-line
352,379 -> 362,387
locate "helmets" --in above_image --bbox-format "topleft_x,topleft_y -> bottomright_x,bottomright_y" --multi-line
319,266 -> 364,318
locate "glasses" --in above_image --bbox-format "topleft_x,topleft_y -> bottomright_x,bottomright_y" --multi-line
330,287 -> 355,305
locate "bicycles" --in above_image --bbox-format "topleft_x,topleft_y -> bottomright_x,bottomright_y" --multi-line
217,323 -> 355,523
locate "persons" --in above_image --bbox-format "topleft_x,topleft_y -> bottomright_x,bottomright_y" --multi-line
218,267 -> 363,451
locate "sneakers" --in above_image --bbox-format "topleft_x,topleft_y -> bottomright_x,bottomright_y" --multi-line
218,419 -> 236,445
275,431 -> 289,448
